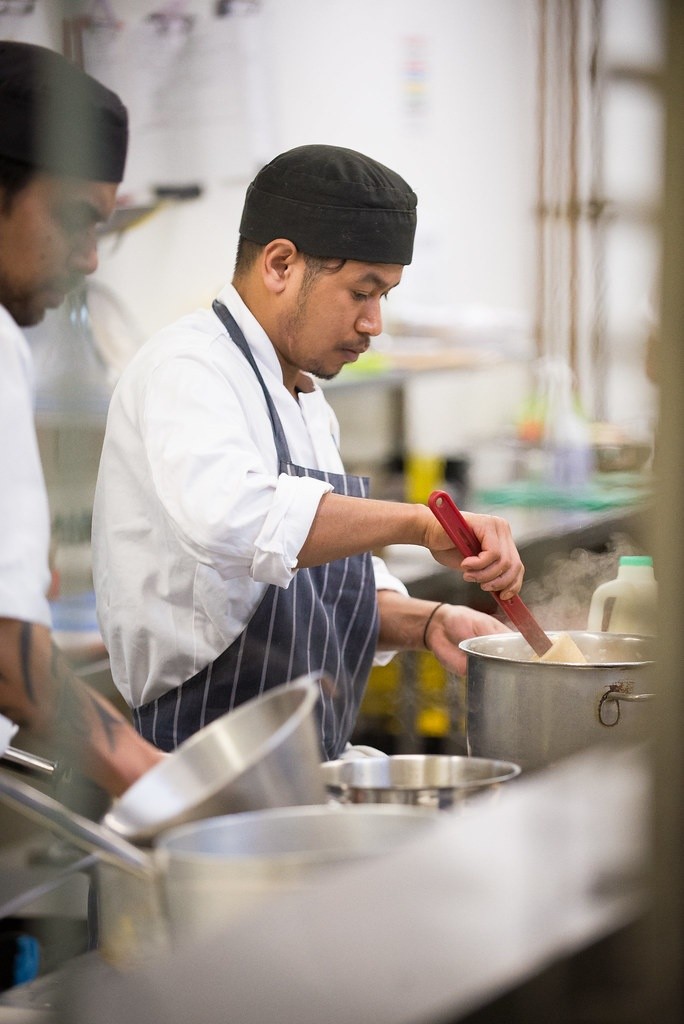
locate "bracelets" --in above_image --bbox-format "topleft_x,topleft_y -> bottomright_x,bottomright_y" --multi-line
422,600 -> 447,653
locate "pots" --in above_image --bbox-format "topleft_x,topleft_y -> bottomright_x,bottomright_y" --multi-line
460,631 -> 657,777
318,757 -> 522,811
153,806 -> 464,893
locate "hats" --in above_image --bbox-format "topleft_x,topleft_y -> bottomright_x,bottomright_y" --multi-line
239,144 -> 417,265
0,40 -> 130,181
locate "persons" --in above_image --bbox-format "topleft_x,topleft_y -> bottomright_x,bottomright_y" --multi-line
92,143 -> 526,786
0,36 -> 177,994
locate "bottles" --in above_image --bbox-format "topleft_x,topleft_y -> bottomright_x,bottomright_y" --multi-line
584,555 -> 659,634
